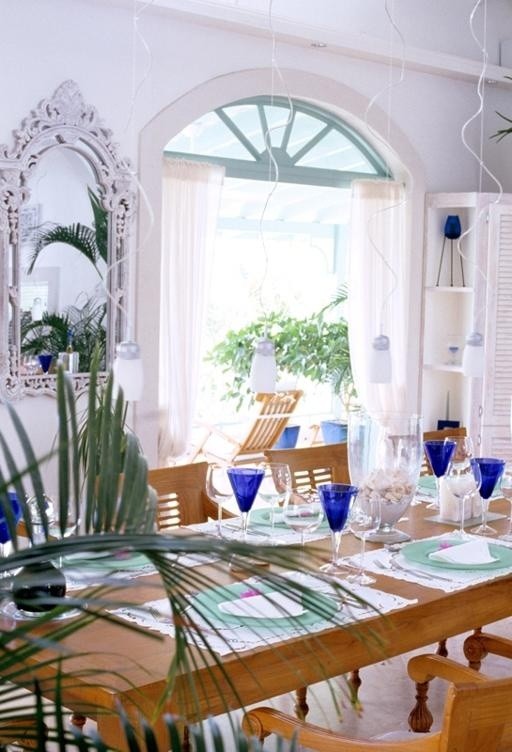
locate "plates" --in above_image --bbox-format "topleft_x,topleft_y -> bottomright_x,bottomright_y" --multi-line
1,594 -> 91,623
401,539 -> 512,570
58,547 -> 168,567
416,474 -> 509,493
191,583 -> 340,629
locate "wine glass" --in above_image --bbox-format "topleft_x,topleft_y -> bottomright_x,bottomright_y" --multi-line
202,461 -> 326,566
1,492 -> 28,581
315,483 -> 383,587
42,491 -> 82,567
447,334 -> 461,365
38,353 -> 53,374
423,434 -> 505,543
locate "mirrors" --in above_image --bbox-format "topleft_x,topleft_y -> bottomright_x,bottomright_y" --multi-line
1,77 -> 142,405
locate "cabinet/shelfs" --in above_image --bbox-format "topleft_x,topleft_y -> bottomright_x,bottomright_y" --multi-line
416,191 -> 511,458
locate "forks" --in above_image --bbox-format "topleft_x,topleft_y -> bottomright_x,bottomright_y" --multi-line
371,556 -> 451,583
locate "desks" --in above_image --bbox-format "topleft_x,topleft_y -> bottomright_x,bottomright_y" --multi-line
0,494 -> 512,750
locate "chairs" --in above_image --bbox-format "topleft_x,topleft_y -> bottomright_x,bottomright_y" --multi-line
78,460 -> 210,529
239,622 -> 512,752
264,444 -> 353,504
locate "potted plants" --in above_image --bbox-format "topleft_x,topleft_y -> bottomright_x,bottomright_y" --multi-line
203,300 -> 302,448
301,281 -> 363,445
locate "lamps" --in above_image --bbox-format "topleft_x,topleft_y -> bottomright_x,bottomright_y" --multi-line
366,0 -> 417,390
247,0 -> 291,394
455,0 -> 508,381
101,0 -> 154,401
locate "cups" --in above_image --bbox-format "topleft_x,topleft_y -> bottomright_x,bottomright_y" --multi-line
347,407 -> 423,543
